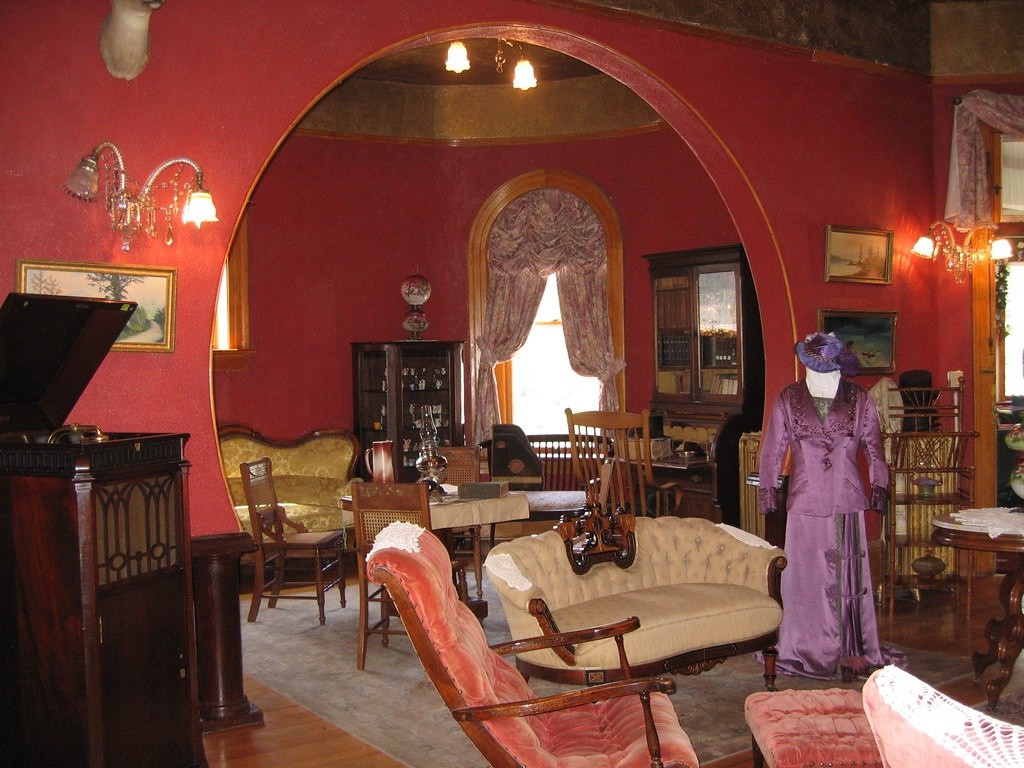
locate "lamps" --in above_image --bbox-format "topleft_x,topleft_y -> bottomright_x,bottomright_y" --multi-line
445,37 -> 539,92
911,221 -> 1014,284
64,142 -> 220,253
101,0 -> 167,81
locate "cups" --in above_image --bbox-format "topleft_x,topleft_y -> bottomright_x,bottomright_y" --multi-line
434,418 -> 441,427
365,440 -> 394,484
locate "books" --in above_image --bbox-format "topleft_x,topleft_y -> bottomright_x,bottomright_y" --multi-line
658,335 -> 690,367
711,374 -> 738,395
458,481 -> 509,498
674,371 -> 693,393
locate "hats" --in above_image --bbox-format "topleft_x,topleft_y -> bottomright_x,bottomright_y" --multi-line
795,332 -> 861,375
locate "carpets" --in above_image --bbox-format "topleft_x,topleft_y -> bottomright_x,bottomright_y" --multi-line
242,572 -> 994,768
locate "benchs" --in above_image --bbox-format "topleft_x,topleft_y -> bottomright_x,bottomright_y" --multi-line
477,435 -> 613,549
484,517 -> 789,691
218,425 -> 361,577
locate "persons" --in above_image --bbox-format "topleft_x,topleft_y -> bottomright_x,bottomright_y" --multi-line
758,332 -> 910,679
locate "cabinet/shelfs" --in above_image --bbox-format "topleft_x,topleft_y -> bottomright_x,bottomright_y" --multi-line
603,244 -> 765,528
882,377 -> 980,622
350,340 -> 467,484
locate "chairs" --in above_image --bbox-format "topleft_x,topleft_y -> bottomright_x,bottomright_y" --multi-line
365,521 -> 699,768
433,446 -> 483,600
565,408 -> 684,518
241,456 -> 347,625
351,482 -> 468,671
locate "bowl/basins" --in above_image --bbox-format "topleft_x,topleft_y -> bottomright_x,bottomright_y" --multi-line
678,452 -> 696,459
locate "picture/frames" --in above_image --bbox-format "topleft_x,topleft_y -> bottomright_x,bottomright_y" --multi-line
17,258 -> 178,353
817,224 -> 899,375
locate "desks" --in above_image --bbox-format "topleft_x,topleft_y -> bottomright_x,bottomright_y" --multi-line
931,507 -> 1024,711
340,484 -> 530,621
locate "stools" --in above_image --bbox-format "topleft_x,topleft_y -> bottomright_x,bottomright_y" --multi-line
746,664 -> 884,768
